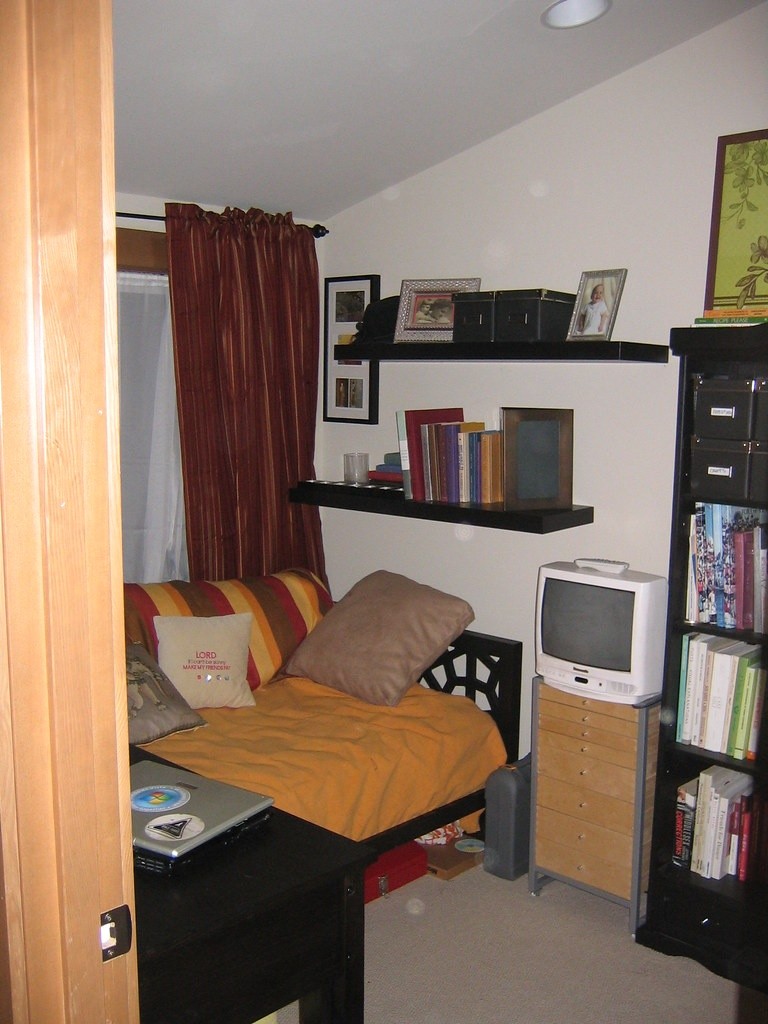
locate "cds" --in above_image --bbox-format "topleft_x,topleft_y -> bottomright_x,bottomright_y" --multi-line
454,840 -> 485,853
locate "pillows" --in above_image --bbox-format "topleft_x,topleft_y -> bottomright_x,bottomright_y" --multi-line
126,644 -> 211,746
153,612 -> 259,708
285,569 -> 475,704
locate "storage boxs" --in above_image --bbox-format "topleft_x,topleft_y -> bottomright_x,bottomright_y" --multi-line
450,288 -> 575,343
423,833 -> 484,882
365,841 -> 427,900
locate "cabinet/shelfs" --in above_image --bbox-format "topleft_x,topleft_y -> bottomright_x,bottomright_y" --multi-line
633,323 -> 767,996
286,341 -> 669,535
532,676 -> 661,937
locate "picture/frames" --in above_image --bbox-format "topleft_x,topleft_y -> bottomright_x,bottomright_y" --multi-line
323,274 -> 380,425
565,269 -> 629,341
704,128 -> 768,309
393,278 -> 482,343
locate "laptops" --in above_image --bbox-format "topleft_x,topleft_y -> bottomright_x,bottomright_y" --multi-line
129,759 -> 274,871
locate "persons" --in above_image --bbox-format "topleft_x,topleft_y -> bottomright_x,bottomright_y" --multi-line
577,285 -> 608,334
337,294 -> 363,322
340,378 -> 359,407
414,298 -> 453,325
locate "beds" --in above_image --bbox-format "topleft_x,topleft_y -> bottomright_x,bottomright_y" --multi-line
123,567 -> 526,865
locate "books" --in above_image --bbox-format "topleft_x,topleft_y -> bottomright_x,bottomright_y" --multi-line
367,407 -> 573,511
672,765 -> 756,882
691,309 -> 768,327
676,631 -> 765,761
684,502 -> 768,635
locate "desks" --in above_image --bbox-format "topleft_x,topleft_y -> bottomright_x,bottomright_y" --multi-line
128,744 -> 365,1024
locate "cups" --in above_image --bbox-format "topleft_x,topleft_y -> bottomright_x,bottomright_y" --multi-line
344,452 -> 370,485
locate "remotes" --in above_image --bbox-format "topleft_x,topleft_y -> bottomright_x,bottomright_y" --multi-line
574,558 -> 629,574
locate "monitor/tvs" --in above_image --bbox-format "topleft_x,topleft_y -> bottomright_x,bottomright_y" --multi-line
534,561 -> 668,705
